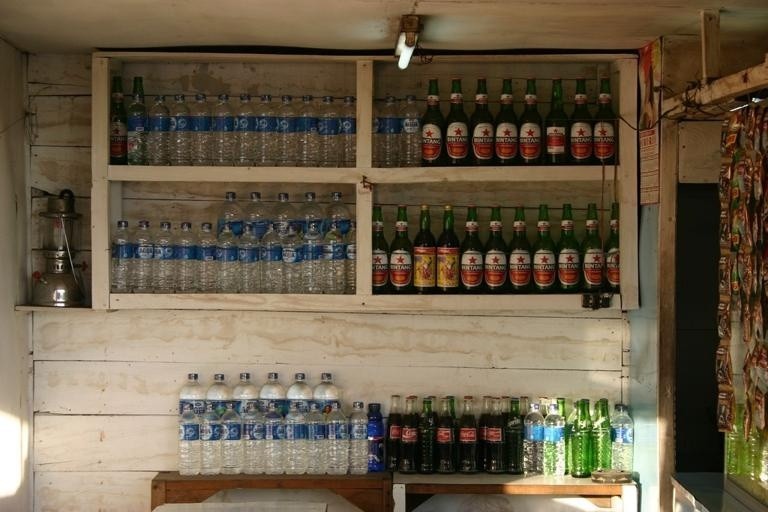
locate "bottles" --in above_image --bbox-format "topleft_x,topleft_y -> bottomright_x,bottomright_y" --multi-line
110,190 -> 354,293
373,74 -> 618,167
177,371 -> 635,484
112,73 -> 357,167
724,402 -> 768,487
370,201 -> 619,293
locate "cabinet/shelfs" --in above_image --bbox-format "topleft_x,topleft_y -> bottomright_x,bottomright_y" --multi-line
85,43 -> 643,311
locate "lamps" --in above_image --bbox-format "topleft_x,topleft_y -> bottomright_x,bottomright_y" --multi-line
392,13 -> 425,69
